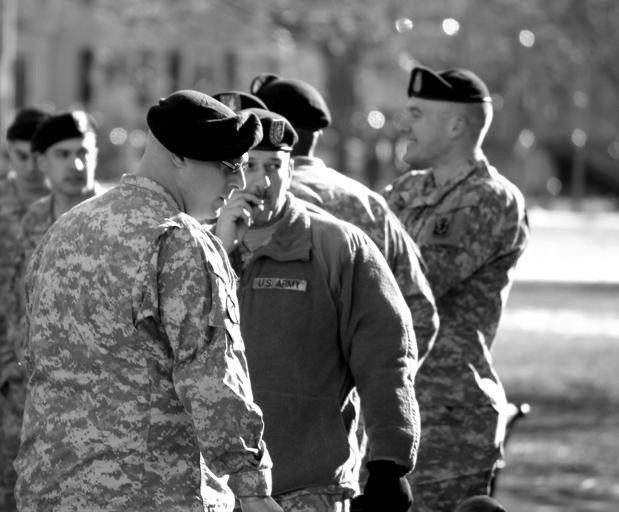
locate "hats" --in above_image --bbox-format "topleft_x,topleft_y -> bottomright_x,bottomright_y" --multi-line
408,68 -> 492,103
7,110 -> 89,152
146,75 -> 331,162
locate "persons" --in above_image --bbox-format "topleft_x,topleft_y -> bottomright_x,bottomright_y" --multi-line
209,87 -> 272,112
1,108 -> 103,510
250,77 -> 444,472
375,64 -> 533,512
1,100 -> 55,298
210,105 -> 426,512
14,87 -> 288,512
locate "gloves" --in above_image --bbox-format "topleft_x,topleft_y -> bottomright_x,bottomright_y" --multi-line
350,460 -> 413,512
